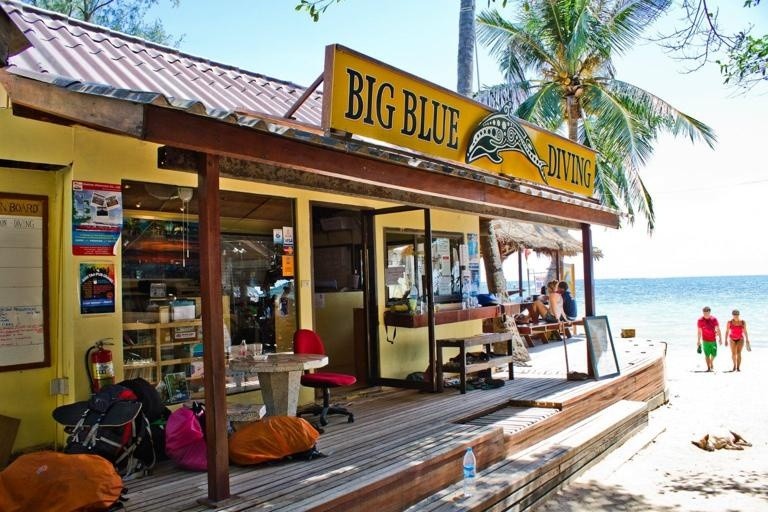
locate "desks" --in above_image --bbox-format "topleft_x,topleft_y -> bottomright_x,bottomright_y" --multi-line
230,352 -> 330,417
149,296 -> 201,319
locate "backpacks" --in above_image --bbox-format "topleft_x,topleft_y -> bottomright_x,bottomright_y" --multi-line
228,415 -> 327,468
52,376 -> 208,479
0,451 -> 129,512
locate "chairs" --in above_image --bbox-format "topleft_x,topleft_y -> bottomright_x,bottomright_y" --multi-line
291,328 -> 357,427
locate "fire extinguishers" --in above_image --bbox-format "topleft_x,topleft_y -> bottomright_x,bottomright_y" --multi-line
85,338 -> 119,394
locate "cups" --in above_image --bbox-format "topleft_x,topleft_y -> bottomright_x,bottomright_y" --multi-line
408,299 -> 417,312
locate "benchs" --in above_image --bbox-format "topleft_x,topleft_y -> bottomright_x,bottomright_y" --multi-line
514,317 -> 585,347
435,332 -> 514,395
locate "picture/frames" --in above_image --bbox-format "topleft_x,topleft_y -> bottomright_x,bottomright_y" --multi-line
77,261 -> 117,318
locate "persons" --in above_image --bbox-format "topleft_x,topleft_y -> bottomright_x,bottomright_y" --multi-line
724,310 -> 750,372
696,306 -> 723,372
517,280 -> 577,324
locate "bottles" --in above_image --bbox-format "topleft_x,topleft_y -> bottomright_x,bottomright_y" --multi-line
240,339 -> 247,360
410,284 -> 418,300
461,281 -> 479,309
462,446 -> 476,497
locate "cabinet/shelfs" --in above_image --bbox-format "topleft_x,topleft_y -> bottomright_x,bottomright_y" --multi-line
122,319 -> 204,399
